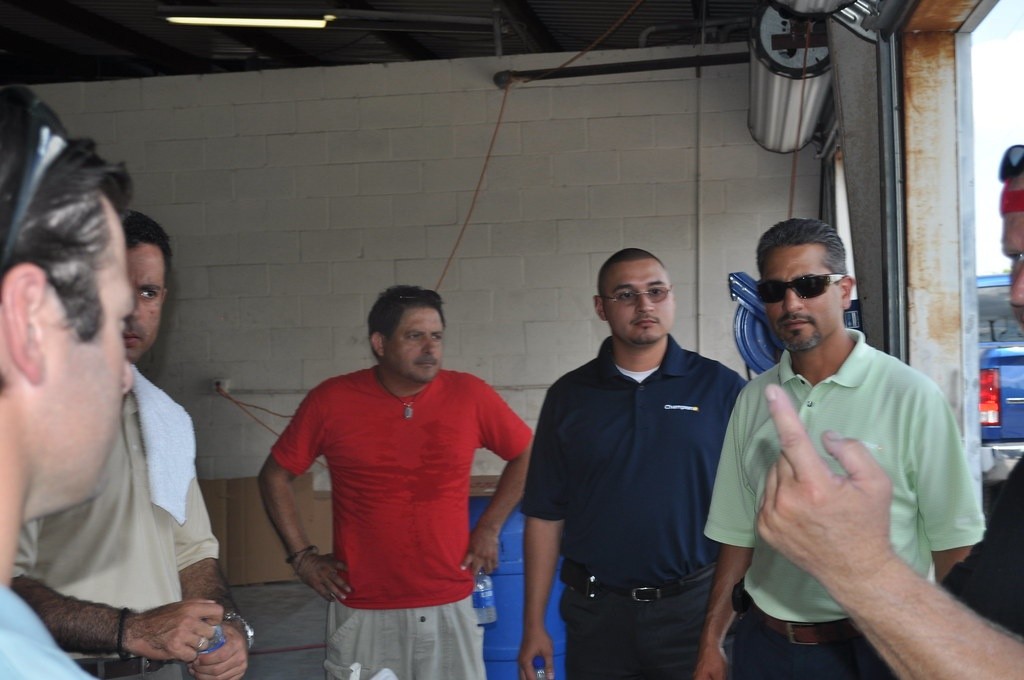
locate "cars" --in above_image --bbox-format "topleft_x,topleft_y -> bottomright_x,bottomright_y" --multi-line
976,273 -> 1023,486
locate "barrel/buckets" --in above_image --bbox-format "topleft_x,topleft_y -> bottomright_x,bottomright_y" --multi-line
469,494 -> 568,680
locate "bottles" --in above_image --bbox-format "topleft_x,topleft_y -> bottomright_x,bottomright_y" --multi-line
197,617 -> 225,655
473,568 -> 497,625
533,657 -> 547,680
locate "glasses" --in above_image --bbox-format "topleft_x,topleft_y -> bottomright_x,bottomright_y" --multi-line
756,274 -> 844,302
999,145 -> 1024,180
383,290 -> 441,303
601,285 -> 673,306
1,84 -> 68,274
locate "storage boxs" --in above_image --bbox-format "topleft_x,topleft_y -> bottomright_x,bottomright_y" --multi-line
194,476 -> 569,680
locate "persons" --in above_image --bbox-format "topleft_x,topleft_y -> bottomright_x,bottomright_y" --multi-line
521,248 -> 747,680
0,83 -> 249,680
695,218 -> 987,680
757,145 -> 1024,680
259,285 -> 534,680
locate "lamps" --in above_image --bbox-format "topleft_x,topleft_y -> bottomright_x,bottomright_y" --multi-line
157,5 -> 507,38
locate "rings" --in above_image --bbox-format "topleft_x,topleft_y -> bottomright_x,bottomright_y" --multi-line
195,637 -> 205,649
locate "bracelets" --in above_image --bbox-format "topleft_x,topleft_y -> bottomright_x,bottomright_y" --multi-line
222,608 -> 255,648
117,607 -> 131,659
286,545 -> 319,574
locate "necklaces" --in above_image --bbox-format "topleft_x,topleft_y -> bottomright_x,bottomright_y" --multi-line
375,364 -> 420,418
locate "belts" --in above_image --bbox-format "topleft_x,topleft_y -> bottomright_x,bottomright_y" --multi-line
599,562 -> 716,601
81,655 -> 177,678
755,606 -> 858,645
